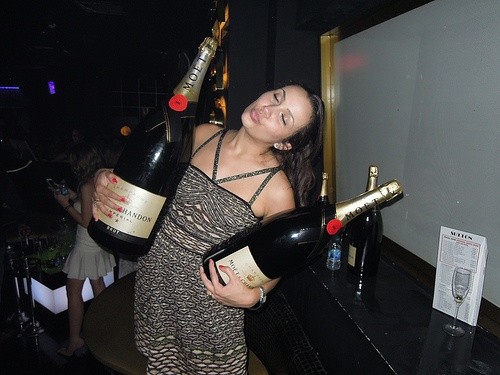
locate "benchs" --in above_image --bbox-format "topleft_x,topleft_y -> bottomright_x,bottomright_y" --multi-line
83,269 -> 329,375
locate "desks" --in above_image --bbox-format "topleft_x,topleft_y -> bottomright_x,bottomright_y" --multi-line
31,270 -> 115,324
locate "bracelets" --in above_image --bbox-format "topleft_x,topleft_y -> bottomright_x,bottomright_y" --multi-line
64,204 -> 73,209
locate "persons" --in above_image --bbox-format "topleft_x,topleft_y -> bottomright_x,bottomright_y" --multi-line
48,145 -> 116,358
90,84 -> 326,375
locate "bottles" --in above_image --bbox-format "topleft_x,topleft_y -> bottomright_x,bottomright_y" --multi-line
347,166 -> 383,280
59,178 -> 68,196
87,37 -> 219,262
326,234 -> 342,271
317,172 -> 330,208
202,178 -> 404,289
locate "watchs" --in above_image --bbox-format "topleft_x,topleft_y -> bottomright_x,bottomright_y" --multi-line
249,286 -> 267,310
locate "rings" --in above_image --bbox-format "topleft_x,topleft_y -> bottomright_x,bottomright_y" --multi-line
207,293 -> 212,300
93,199 -> 100,205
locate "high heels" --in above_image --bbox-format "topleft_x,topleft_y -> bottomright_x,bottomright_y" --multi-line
57,340 -> 86,356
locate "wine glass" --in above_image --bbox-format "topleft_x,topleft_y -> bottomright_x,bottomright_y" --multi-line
444,267 -> 472,337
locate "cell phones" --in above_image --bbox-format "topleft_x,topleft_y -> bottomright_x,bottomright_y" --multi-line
46,178 -> 61,191
